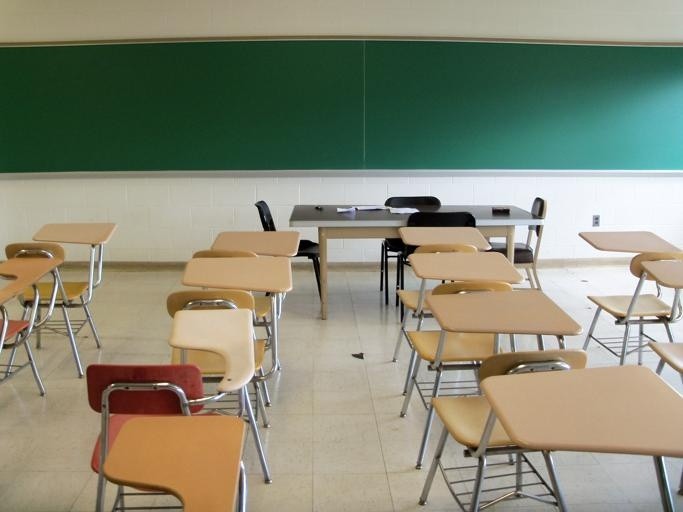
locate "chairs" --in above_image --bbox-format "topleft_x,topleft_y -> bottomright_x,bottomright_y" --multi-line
254,200 -> 324,301
379,195 -> 440,305
395,211 -> 475,323
484,196 -> 546,293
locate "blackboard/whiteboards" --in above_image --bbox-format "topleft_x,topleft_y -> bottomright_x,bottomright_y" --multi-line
0,38 -> 683,174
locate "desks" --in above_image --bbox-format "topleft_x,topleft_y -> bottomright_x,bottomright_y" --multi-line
392,226 -> 683,512
84,231 -> 302,511
0,219 -> 116,397
288,204 -> 545,319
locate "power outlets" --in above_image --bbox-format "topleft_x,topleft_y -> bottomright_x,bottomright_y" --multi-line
593,215 -> 600,227
